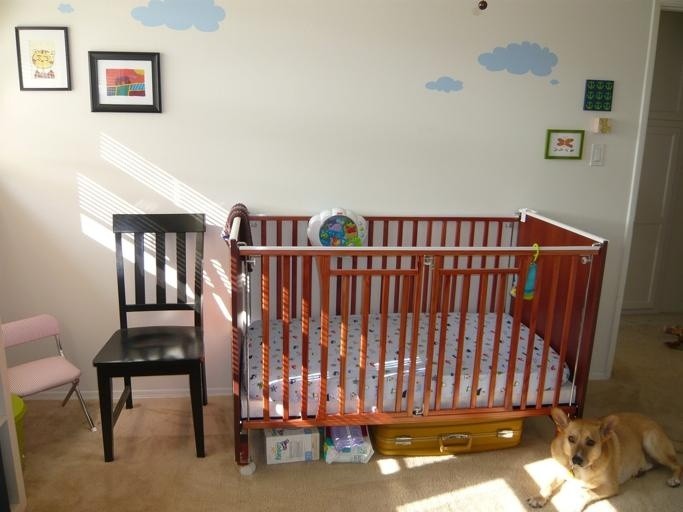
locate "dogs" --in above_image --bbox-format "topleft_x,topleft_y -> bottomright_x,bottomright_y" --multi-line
521,404 -> 683,512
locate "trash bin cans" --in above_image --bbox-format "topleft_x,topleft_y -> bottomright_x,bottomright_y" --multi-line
10,394 -> 27,473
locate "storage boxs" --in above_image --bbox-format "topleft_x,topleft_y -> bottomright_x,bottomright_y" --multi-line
263,429 -> 320,464
323,425 -> 374,464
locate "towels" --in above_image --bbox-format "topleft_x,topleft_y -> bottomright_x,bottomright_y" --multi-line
220,204 -> 254,273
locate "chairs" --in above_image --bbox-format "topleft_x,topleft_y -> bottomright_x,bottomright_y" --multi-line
2,313 -> 98,432
93,214 -> 209,462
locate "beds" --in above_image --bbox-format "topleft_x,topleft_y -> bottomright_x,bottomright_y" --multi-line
229,209 -> 607,470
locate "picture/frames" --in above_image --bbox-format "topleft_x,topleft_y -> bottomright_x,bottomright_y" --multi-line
88,50 -> 162,113
543,129 -> 585,160
14,27 -> 71,91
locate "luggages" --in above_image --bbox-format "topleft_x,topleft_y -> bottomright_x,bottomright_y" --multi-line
374,418 -> 522,456
